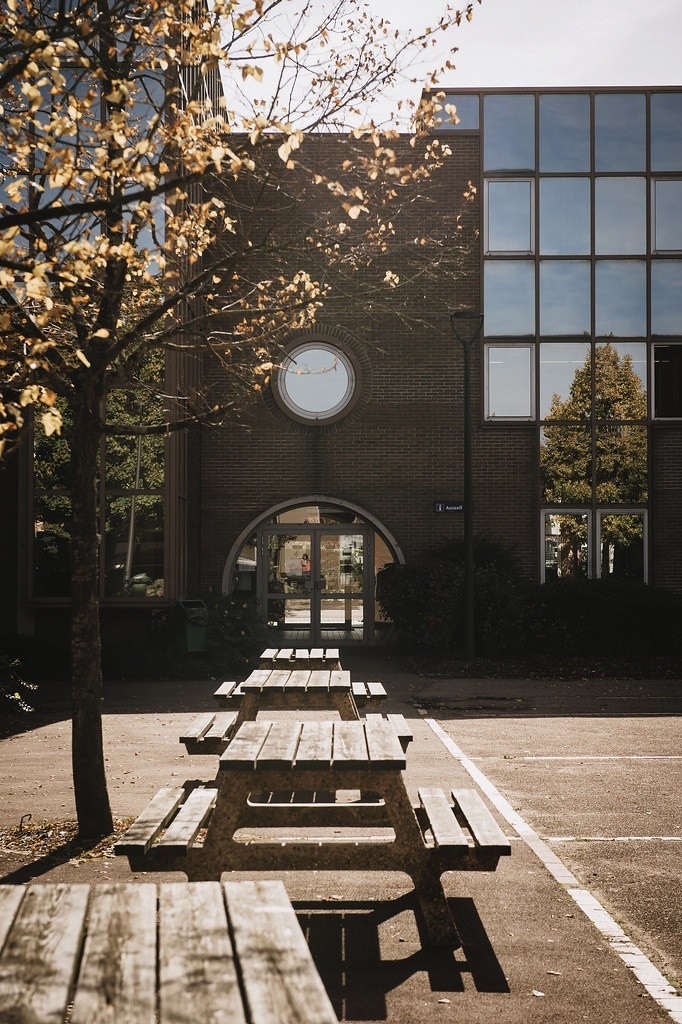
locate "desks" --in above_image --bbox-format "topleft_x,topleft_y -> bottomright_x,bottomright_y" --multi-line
0,880 -> 338,1024
259,649 -> 343,671
229,669 -> 361,739
188,721 -> 462,950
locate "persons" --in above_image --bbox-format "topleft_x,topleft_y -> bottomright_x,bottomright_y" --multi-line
301,554 -> 310,577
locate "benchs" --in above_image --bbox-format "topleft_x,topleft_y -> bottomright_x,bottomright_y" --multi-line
114,680 -> 512,873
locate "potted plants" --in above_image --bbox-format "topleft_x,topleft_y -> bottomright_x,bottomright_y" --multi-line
130,572 -> 152,597
